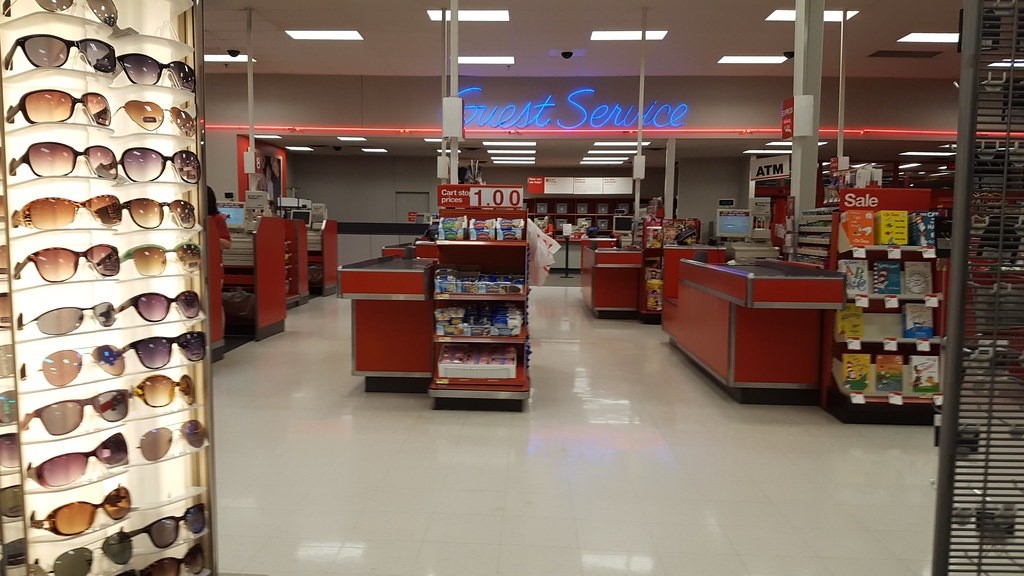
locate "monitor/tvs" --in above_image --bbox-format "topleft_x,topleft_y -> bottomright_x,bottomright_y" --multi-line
613,216 -> 633,234
216,201 -> 245,228
291,210 -> 311,227
717,209 -> 752,237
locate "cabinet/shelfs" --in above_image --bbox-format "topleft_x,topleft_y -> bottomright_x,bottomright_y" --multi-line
828,187 -> 943,425
793,208 -> 832,270
527,198 -> 634,237
638,218 -> 703,324
429,210 -> 531,400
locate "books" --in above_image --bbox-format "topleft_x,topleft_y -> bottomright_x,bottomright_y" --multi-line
833,209 -> 941,393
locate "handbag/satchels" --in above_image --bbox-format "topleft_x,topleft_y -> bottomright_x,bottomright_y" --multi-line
528,219 -> 562,285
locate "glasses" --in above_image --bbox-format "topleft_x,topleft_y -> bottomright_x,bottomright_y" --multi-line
0,0 -> 206,576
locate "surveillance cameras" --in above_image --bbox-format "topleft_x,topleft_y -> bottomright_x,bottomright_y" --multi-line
333,146 -> 342,151
227,50 -> 240,57
783,52 -> 794,59
562,52 -> 573,59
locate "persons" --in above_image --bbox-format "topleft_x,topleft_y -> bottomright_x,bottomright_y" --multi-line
646,218 -> 669,308
206,183 -> 234,345
257,153 -> 281,217
